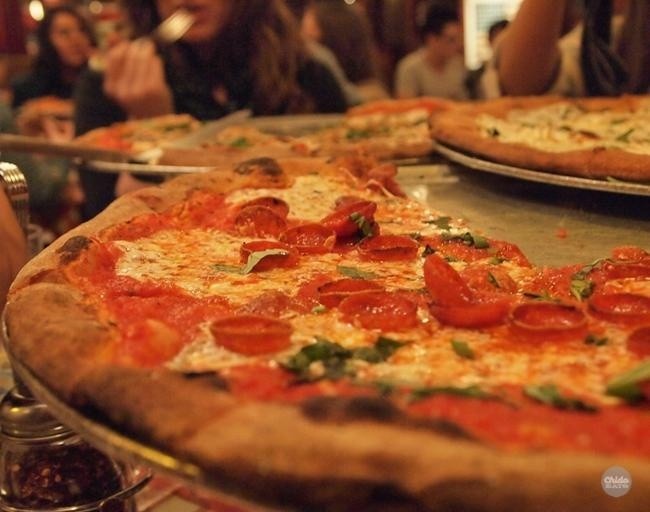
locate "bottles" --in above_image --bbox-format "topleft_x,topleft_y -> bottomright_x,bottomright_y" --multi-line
0,382 -> 139,512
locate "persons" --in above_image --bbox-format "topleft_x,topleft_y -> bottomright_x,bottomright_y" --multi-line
0,0 -> 650,316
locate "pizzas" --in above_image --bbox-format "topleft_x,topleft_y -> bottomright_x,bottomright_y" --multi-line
71,95 -> 451,168
1,153 -> 650,511
429,93 -> 650,182
